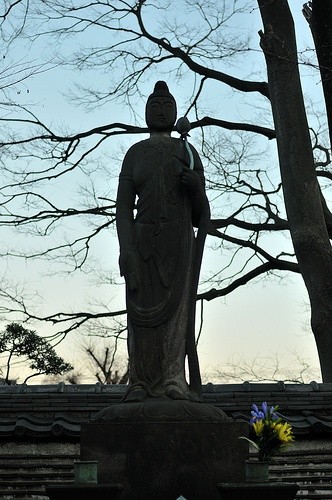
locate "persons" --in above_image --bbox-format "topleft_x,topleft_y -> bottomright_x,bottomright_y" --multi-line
111,80 -> 215,404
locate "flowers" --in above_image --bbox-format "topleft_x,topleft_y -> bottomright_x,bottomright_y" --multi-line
249,402 -> 295,461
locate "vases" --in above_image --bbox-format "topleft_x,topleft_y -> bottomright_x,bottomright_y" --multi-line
72,459 -> 99,484
244,460 -> 271,484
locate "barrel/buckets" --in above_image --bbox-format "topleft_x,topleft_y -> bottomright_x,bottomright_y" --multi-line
74,460 -> 98,484
245,460 -> 269,484
